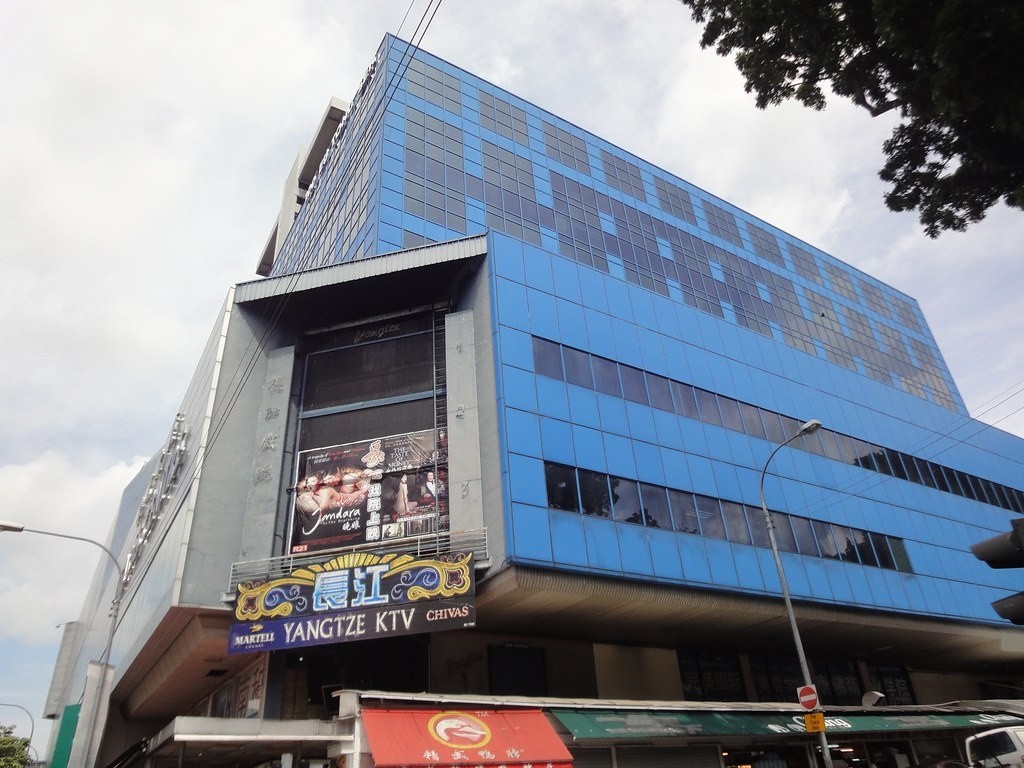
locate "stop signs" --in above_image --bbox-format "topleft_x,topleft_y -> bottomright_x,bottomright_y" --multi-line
796,684 -> 820,711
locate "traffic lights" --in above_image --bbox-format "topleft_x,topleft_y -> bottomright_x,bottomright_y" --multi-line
969,518 -> 1024,626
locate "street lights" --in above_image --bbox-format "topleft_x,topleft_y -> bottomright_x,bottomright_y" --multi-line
760,419 -> 835,768
0,522 -> 123,768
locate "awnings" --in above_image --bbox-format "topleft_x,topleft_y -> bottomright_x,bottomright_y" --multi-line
357,706 -> 576,768
550,709 -> 1024,742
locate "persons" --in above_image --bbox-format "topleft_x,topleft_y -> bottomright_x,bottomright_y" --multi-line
419,471 -> 446,498
391,472 -> 418,514
384,525 -> 392,538
298,465 -> 368,510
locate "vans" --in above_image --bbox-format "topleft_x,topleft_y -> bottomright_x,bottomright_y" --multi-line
965,726 -> 1024,768
